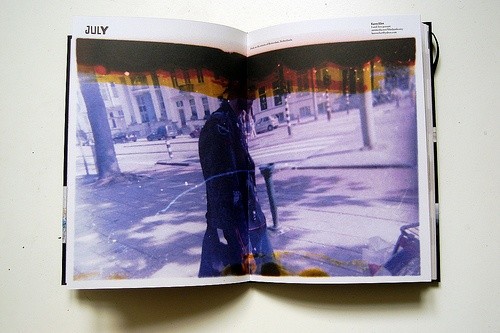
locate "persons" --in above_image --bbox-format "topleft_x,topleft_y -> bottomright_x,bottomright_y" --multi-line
197,74 -> 280,280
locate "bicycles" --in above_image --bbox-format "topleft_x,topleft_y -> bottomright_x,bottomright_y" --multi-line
372,221 -> 421,276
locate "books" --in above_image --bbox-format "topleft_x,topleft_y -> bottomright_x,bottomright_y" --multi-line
57,13 -> 443,295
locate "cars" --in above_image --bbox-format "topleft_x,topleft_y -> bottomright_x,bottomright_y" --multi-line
253,115 -> 279,132
146,124 -> 177,141
190,125 -> 203,138
111,130 -> 137,143
